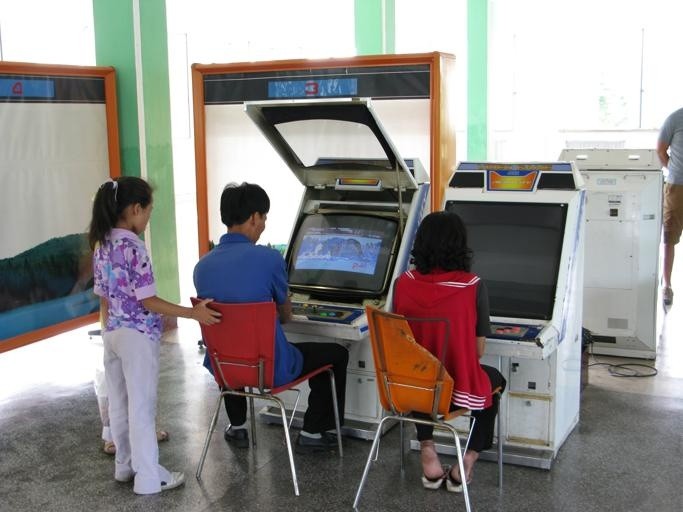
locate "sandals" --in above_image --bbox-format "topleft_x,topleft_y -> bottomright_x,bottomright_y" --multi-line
156,428 -> 169,441
104,440 -> 117,454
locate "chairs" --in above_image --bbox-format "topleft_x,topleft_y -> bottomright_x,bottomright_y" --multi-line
189,296 -> 345,496
352,304 -> 503,512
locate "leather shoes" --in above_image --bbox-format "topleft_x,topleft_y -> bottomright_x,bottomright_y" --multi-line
296,433 -> 343,453
224,425 -> 250,448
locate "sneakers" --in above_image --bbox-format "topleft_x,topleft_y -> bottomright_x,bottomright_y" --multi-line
662,281 -> 673,304
161,472 -> 186,490
422,462 -> 451,487
115,469 -> 134,482
446,464 -> 474,493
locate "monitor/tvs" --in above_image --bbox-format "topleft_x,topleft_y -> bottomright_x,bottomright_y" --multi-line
283,209 -> 408,295
440,200 -> 569,319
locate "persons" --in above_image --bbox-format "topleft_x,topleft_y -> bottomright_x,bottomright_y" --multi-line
67,244 -> 167,453
195,182 -> 348,453
656,109 -> 683,305
87,176 -> 221,493
392,212 -> 506,493
